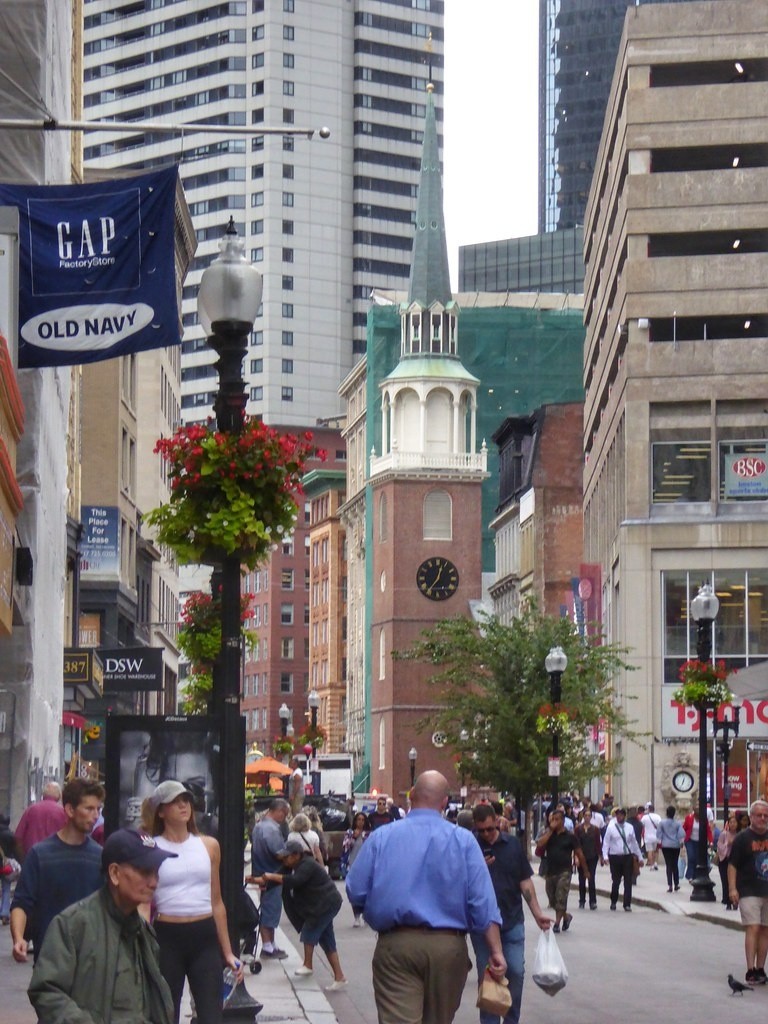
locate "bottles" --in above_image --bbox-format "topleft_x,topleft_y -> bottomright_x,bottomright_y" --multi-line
223,962 -> 240,1010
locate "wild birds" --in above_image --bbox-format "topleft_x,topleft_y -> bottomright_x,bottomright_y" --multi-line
727,974 -> 754,997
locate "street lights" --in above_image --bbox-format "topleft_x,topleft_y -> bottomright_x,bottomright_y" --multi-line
544,641 -> 571,805
308,690 -> 320,794
197,208 -> 269,1023
458,727 -> 470,810
276,702 -> 291,802
407,746 -> 417,790
685,579 -> 722,909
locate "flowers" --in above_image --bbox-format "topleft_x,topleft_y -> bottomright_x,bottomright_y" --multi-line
296,724 -> 332,753
671,660 -> 741,712
177,585 -> 259,717
270,735 -> 297,759
137,409 -> 328,576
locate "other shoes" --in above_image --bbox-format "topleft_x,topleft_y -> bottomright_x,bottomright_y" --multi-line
726,904 -> 731,910
674,885 -> 681,891
624,904 -> 633,913
666,888 -> 673,893
733,905 -> 739,911
553,914 -> 573,933
589,902 -> 598,910
610,901 -> 619,910
578,900 -> 586,910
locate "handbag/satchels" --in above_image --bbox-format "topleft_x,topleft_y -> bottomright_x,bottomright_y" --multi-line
632,857 -> 640,878
3,857 -> 22,882
713,852 -> 719,866
532,927 -> 568,996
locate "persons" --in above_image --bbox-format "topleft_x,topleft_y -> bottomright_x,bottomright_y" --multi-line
26,827 -> 179,1024
601,807 -> 644,911
137,780 -> 245,1024
0,778 -> 105,925
245,840 -> 348,991
10,776 -> 105,971
727,800 -> 768,985
251,799 -> 288,958
655,800 -> 752,911
532,791 -> 662,869
469,803 -> 557,1024
340,797 -> 518,928
344,769 -> 508,1024
537,810 -> 591,932
250,801 -> 329,867
573,807 -> 604,910
289,758 -> 305,816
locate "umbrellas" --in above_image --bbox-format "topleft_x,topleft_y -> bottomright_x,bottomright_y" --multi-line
243,756 -> 295,791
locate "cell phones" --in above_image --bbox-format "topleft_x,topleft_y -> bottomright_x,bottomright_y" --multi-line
482,849 -> 493,858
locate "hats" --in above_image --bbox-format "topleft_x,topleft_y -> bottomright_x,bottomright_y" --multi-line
615,808 -> 626,815
102,830 -> 178,870
148,778 -> 197,813
276,841 -> 303,857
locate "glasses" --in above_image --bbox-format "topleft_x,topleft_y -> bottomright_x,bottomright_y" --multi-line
477,825 -> 495,833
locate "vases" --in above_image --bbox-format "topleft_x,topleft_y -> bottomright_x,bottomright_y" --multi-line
693,699 -> 717,713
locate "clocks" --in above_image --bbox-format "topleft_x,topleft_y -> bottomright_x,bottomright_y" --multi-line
416,557 -> 459,600
672,771 -> 695,793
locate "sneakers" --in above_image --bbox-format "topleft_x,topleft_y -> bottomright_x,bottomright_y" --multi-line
352,919 -> 362,929
295,964 -> 313,977
325,978 -> 349,993
743,967 -> 768,985
260,947 -> 288,960
